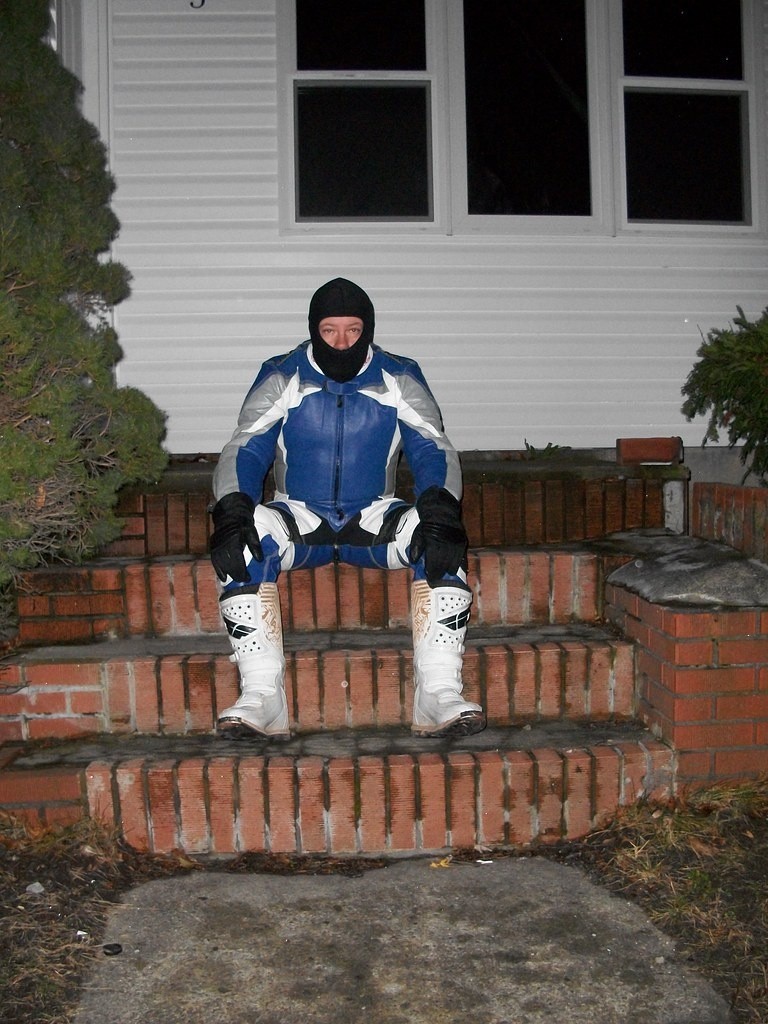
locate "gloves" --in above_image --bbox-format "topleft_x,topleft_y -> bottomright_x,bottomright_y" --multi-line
409,486 -> 469,580
209,492 -> 264,584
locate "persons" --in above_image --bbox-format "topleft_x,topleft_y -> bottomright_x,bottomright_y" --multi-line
207,277 -> 487,744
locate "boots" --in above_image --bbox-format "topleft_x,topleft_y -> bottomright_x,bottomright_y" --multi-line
218,582 -> 291,742
410,578 -> 486,737
